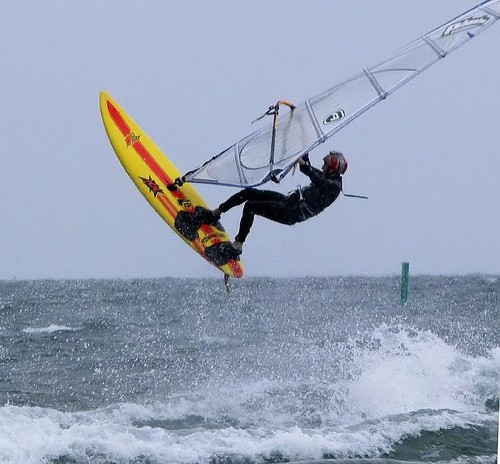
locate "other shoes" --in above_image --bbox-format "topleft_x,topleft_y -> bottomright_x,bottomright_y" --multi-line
217,240 -> 242,257
195,205 -> 222,224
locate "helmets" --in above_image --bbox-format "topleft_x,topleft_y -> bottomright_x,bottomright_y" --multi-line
322,149 -> 348,174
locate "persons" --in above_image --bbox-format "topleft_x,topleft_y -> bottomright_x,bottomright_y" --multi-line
195,150 -> 348,259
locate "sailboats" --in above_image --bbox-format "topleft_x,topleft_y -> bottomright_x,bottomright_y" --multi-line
98,1 -> 498,279
